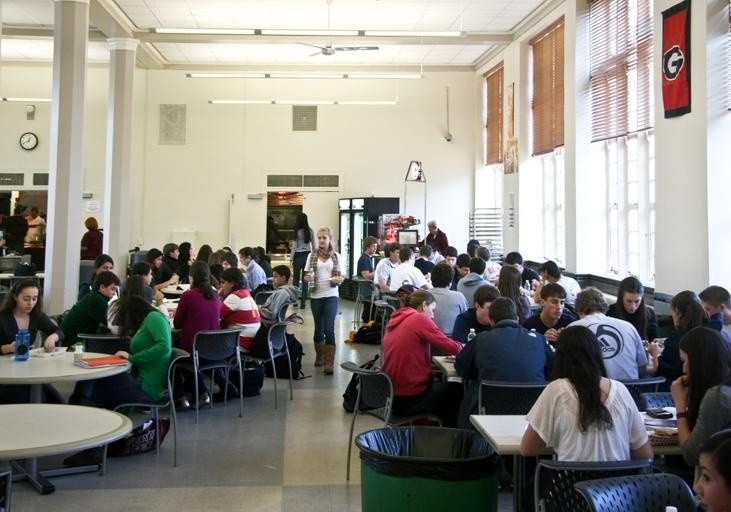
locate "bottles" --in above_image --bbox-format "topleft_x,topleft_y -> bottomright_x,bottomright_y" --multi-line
466,328 -> 477,346
73,342 -> 84,366
308,267 -> 315,289
523,279 -> 530,301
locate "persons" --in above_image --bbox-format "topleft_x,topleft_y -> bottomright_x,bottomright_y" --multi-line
669,325 -> 731,494
520,325 -> 656,470
266,215 -> 282,255
646,291 -> 731,377
291,213 -> 318,310
699,283 -> 731,337
3,204 -> 32,257
607,274 -> 663,339
302,225 -> 348,377
357,217 -> 586,356
453,298 -> 557,415
693,429 -> 731,511
563,285 -> 650,384
380,289 -> 468,428
79,216 -> 104,258
24,206 -> 46,247
1,277 -> 67,359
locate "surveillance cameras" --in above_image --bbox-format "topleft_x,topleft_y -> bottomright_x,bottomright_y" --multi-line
443,133 -> 453,142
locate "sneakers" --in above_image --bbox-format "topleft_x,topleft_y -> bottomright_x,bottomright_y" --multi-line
175,391 -> 210,411
60,450 -> 106,468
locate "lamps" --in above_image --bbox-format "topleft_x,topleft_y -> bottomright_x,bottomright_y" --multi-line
2,95 -> 54,103
148,25 -> 469,106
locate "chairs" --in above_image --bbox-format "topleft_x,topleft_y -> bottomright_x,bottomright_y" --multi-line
96,346 -> 191,476
172,325 -> 247,425
341,254 -> 731,511
1,256 -> 304,409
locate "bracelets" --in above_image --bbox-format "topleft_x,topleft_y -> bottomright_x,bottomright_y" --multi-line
675,408 -> 689,422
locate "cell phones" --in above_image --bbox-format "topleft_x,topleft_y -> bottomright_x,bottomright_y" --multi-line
647,408 -> 673,419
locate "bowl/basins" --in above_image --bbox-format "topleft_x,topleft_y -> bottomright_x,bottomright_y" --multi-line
331,277 -> 343,283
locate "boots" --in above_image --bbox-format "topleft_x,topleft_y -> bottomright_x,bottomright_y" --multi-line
311,338 -> 337,375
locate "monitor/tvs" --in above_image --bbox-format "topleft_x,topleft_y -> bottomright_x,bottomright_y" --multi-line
397,230 -> 418,247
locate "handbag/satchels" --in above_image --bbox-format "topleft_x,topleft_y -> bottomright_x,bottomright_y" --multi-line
112,415 -> 172,458
342,357 -> 392,414
229,358 -> 266,397
353,322 -> 383,344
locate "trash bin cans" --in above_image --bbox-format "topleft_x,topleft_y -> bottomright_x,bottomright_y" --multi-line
355,425 -> 503,512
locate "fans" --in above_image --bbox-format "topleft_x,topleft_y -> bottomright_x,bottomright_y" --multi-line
293,0 -> 380,59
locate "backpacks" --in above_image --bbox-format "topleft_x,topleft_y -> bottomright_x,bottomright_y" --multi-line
269,332 -> 309,381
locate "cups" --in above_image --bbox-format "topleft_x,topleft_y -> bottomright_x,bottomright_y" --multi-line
642,340 -> 650,365
13,328 -> 30,362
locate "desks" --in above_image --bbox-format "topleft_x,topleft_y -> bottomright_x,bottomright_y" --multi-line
1,404 -> 133,511
1,348 -> 132,495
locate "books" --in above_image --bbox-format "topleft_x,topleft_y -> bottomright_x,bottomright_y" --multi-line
644,418 -> 679,428
9,344 -> 68,361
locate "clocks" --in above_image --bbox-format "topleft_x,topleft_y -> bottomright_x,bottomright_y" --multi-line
19,131 -> 40,151
